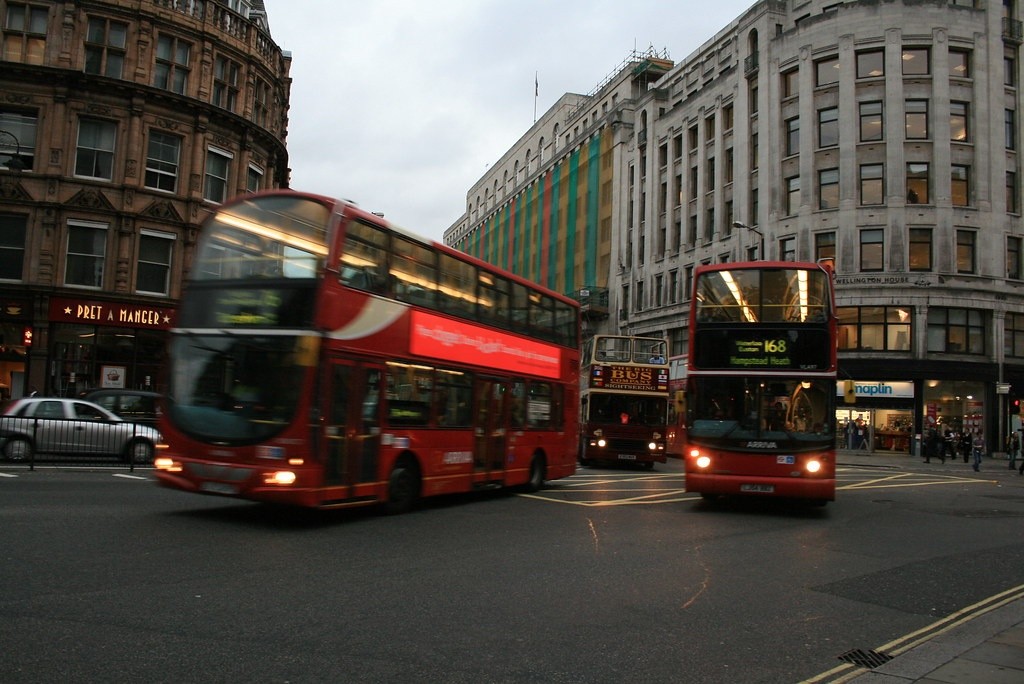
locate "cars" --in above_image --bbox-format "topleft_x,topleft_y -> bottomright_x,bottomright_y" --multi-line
1,397 -> 163,466
85,389 -> 165,443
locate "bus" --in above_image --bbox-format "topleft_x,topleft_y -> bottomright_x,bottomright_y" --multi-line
578,333 -> 670,470
683,262 -> 836,513
150,189 -> 582,520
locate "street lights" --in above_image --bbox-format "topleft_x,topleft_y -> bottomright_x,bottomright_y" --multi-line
731,221 -> 765,440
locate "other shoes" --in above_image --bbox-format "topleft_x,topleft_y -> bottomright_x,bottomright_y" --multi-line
942,459 -> 946,464
1008,467 -> 1016,470
971,465 -> 979,472
923,461 -> 931,463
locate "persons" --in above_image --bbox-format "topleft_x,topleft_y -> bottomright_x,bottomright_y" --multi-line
716,295 -> 741,321
649,349 -> 664,364
765,402 -> 1024,476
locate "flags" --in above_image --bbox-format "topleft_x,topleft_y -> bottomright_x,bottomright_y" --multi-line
535,78 -> 539,96
